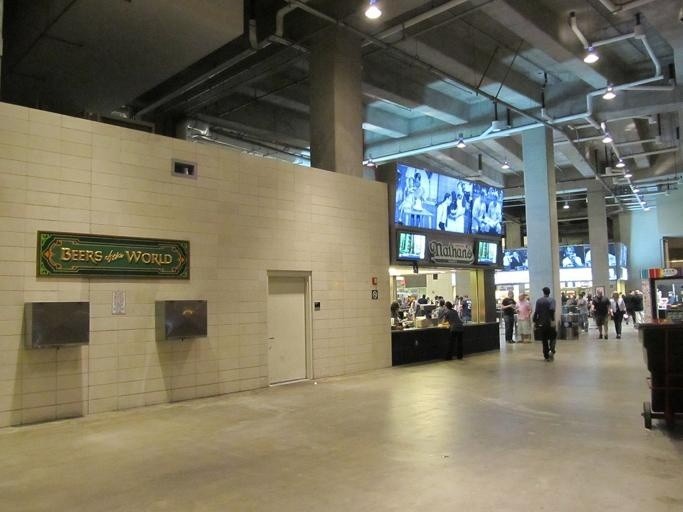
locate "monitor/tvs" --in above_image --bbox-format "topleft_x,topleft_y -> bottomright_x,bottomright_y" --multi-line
396,230 -> 428,262
475,240 -> 499,265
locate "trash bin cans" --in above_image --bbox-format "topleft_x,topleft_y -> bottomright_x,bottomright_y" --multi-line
561,314 -> 579,340
639,323 -> 683,427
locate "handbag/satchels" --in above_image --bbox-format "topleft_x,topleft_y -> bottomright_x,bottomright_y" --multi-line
534,325 -> 544,340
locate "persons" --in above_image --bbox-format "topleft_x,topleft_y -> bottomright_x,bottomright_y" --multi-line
503,252 -> 523,269
439,178 -> 470,235
442,301 -> 464,360
501,288 -> 644,343
400,172 -> 425,231
473,187 -> 504,236
562,247 -> 582,267
533,287 -> 557,361
391,294 -> 472,321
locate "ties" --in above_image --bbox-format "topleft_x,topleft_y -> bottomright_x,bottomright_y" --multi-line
616,301 -> 620,312
